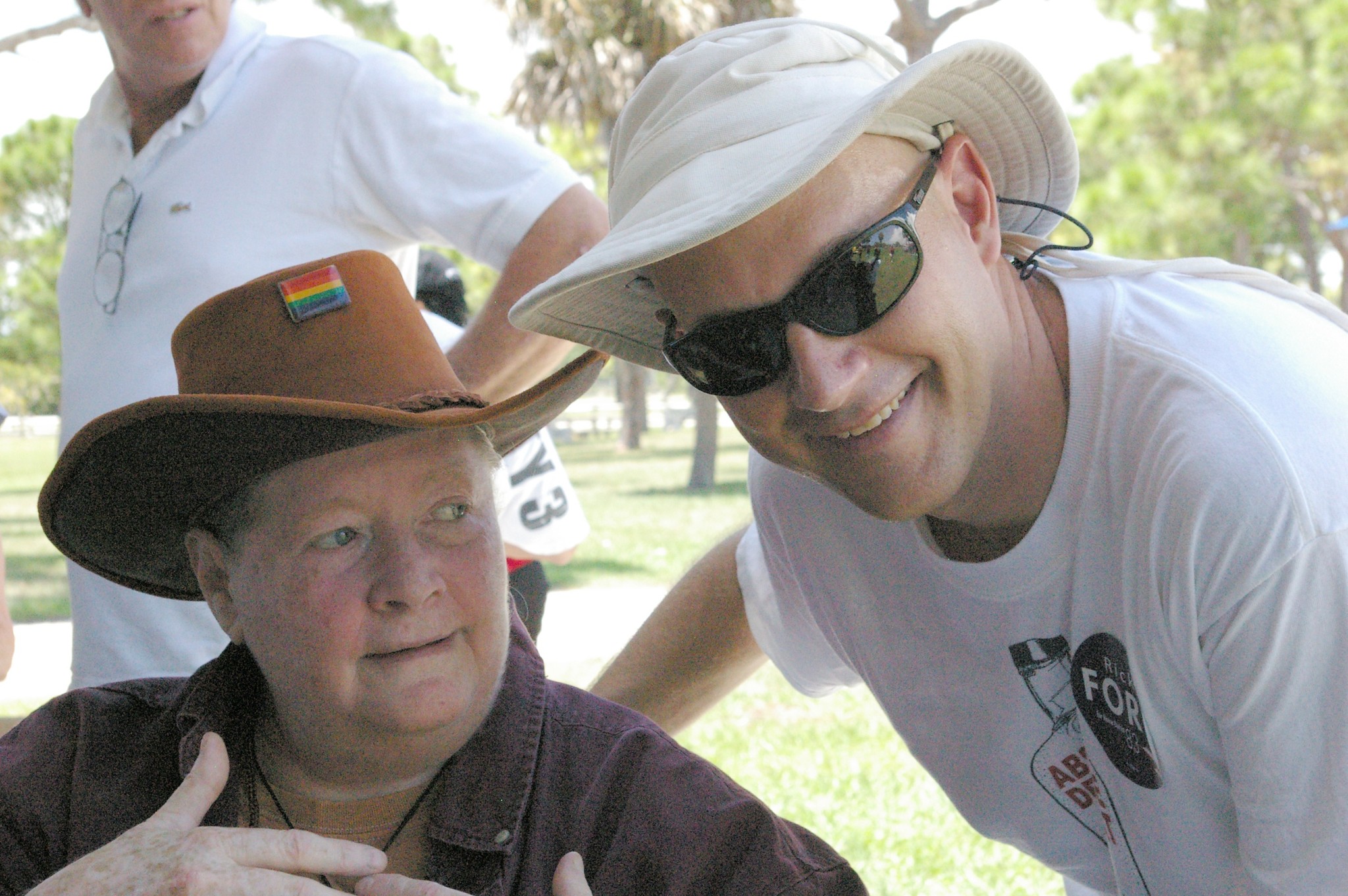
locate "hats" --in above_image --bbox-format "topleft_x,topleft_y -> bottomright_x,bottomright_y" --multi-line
509,15 -> 1078,377
35,250 -> 610,603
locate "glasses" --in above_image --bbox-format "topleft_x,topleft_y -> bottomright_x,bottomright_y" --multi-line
662,143 -> 948,396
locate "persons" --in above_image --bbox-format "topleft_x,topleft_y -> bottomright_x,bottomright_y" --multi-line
510,15 -> 1347,896
56,0 -> 612,691
0,249 -> 878,896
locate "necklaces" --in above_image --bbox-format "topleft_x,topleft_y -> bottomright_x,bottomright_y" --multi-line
247,725 -> 458,890
